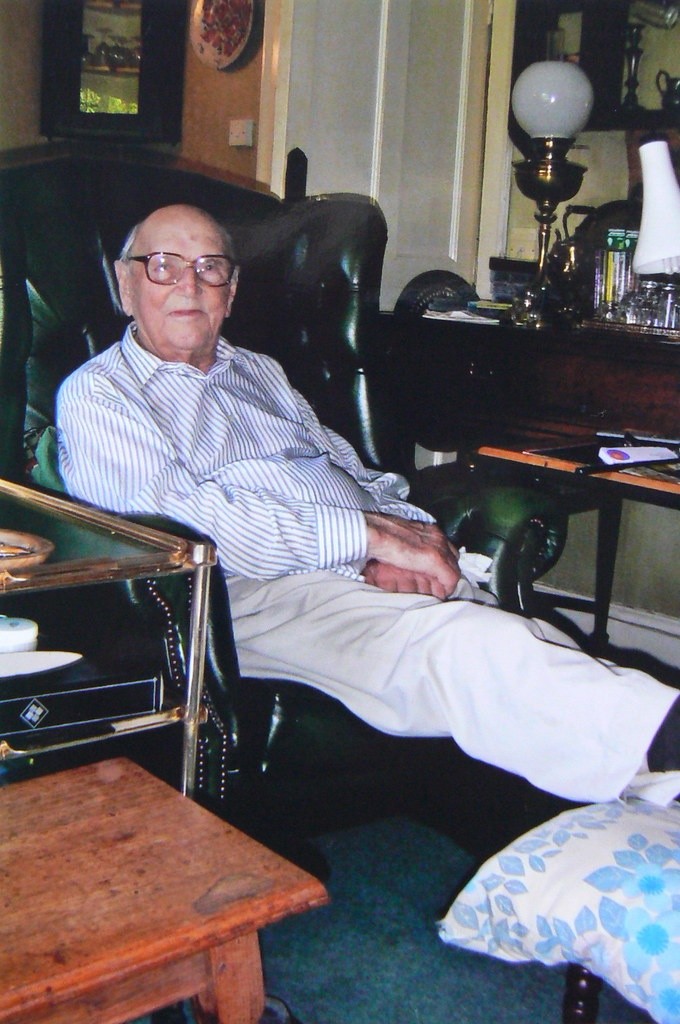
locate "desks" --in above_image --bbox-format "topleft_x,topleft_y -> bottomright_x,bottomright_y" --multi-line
382,316 -> 679,462
475,433 -> 679,643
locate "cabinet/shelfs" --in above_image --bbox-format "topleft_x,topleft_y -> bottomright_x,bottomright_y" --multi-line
506,0 -> 680,132
0,474 -> 218,799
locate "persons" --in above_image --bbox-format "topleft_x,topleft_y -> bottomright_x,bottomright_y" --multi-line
54,203 -> 680,808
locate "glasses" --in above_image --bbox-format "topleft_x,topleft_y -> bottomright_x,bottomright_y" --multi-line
129,253 -> 235,286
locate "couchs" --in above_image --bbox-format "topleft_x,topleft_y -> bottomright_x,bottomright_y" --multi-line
0,154 -> 569,851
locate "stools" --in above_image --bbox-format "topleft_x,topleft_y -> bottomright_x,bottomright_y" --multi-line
1,757 -> 328,1023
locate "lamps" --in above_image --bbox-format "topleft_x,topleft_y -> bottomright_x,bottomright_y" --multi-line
502,60 -> 595,330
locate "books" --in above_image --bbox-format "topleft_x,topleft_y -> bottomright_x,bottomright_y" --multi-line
593,248 -> 635,308
